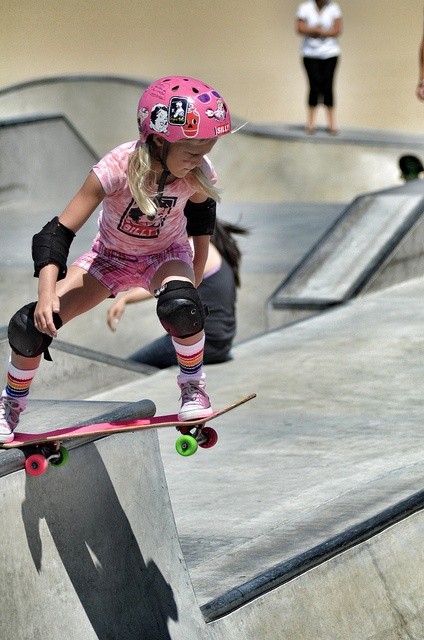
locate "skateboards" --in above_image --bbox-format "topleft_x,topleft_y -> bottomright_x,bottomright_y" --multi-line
0,394 -> 259,475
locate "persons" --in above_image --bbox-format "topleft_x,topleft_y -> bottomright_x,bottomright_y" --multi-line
295,0 -> 344,136
0,75 -> 232,444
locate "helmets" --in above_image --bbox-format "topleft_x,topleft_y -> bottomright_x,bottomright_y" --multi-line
137,76 -> 231,202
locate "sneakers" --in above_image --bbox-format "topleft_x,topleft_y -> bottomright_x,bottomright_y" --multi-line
0,390 -> 28,444
178,381 -> 213,421
